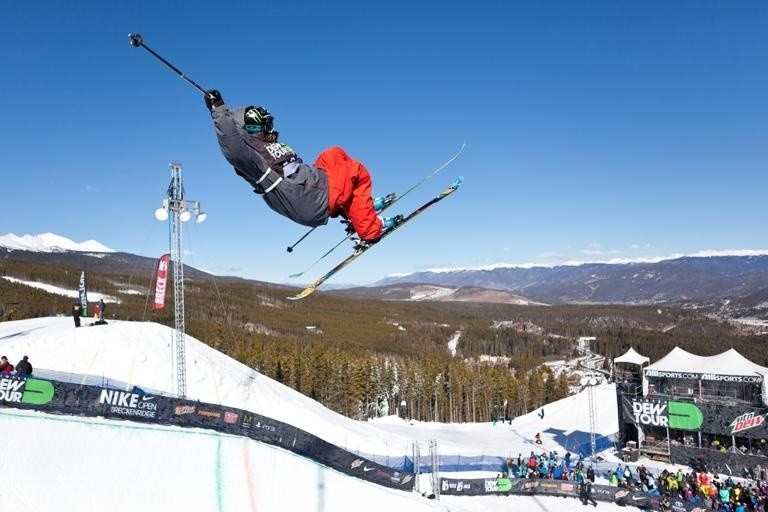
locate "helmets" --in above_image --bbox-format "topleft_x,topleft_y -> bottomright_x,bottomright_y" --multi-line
244,106 -> 274,132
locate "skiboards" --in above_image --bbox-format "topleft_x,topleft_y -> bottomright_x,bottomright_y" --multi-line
286,140 -> 467,300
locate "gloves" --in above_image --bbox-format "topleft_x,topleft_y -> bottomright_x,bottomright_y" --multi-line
204,90 -> 224,109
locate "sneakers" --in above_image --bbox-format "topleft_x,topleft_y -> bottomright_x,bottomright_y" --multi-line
381,217 -> 395,234
371,197 -> 384,210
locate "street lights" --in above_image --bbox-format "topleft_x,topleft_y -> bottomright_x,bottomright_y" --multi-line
580,377 -> 608,461
155,162 -> 208,398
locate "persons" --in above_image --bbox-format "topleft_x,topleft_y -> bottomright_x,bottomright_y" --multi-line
99,299 -> 105,322
73,302 -> 81,328
95,302 -> 100,318
16,356 -> 34,375
0,356 -> 13,375
206,88 -> 402,246
495,432 -> 768,512
